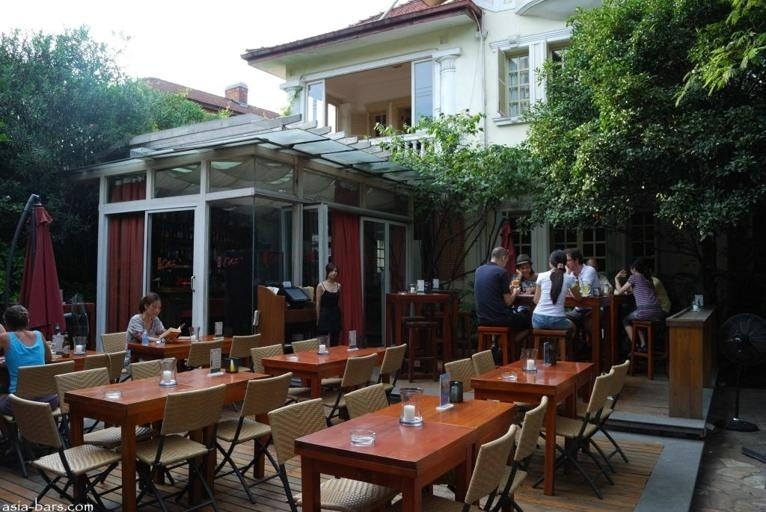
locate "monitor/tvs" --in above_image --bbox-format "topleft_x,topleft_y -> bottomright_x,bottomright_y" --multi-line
280,287 -> 312,310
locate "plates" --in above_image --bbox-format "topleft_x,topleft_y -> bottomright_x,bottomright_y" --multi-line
581,295 -> 591,297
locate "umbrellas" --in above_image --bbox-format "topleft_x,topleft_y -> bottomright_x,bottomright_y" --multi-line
5,196 -> 67,342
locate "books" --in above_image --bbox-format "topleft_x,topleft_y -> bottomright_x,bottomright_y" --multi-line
158,322 -> 187,343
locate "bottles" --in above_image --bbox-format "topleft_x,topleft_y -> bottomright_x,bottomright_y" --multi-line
141,328 -> 149,346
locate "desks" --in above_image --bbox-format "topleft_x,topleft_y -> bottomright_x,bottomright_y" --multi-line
666,304 -> 717,419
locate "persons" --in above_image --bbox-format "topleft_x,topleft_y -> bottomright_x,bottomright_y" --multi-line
470,244 -> 672,369
313,262 -> 344,348
0,305 -> 60,436
117,292 -> 169,383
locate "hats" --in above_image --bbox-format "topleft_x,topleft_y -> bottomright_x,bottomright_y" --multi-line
516,254 -> 530,265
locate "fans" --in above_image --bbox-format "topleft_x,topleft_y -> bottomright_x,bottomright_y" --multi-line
714,313 -> 766,433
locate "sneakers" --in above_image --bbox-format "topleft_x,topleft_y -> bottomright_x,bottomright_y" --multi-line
627,345 -> 647,357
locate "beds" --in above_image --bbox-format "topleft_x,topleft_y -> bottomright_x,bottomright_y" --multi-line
512,294 -> 633,391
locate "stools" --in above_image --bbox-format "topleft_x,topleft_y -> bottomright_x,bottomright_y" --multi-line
401,307 -> 665,384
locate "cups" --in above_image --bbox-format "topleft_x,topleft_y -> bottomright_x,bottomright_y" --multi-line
449,381 -> 464,403
512,276 -> 521,287
400,387 -> 424,425
159,358 -> 177,387
521,349 -> 539,371
582,285 -> 592,294
189,326 -> 200,343
691,298 -> 701,311
408,283 -> 419,294
600,283 -> 612,297
593,287 -> 601,297
72,336 -> 88,355
